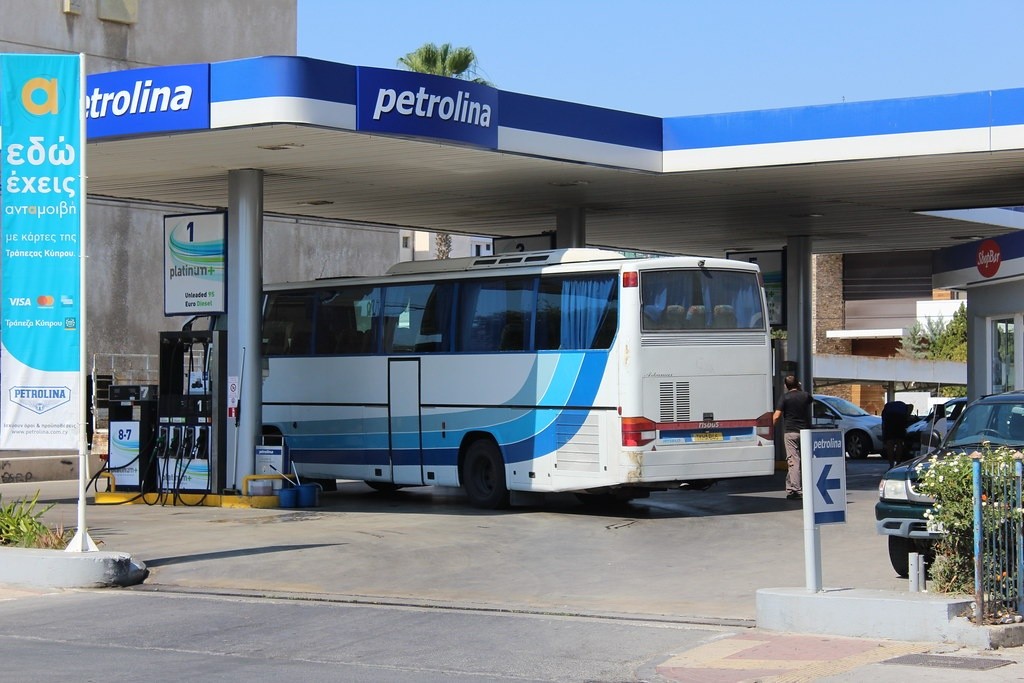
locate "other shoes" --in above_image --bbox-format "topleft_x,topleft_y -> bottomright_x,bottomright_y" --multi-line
787,493 -> 802,500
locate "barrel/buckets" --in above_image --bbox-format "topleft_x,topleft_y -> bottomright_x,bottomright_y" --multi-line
296,484 -> 317,507
278,488 -> 297,509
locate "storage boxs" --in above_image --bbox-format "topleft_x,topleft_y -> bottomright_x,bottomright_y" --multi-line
248,480 -> 272,495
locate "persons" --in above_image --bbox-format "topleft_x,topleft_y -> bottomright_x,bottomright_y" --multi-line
881,401 -> 913,473
772,375 -> 814,500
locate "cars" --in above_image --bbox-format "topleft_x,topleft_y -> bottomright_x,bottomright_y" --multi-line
875,389 -> 1023,576
812,394 -> 883,461
905,397 -> 968,457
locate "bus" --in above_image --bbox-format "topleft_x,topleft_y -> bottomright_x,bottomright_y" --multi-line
180,247 -> 777,513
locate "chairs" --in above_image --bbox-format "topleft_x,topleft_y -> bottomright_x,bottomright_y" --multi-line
712,304 -> 736,328
660,305 -> 686,329
684,305 -> 707,329
644,304 -> 659,330
1009,407 -> 1024,441
